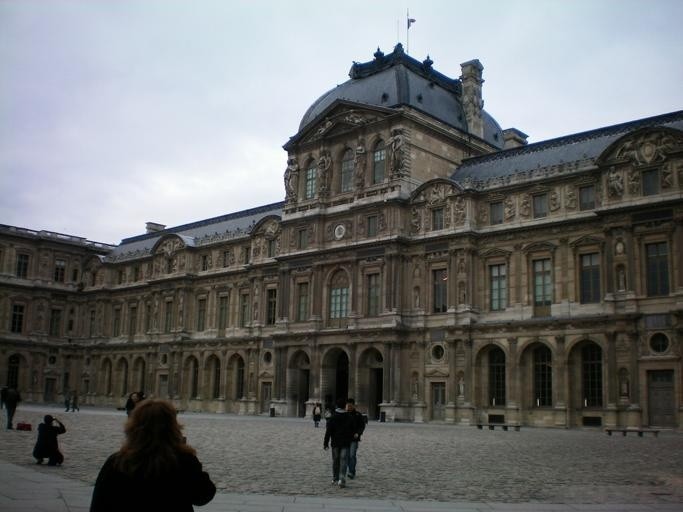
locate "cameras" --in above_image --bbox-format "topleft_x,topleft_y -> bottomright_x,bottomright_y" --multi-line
53,417 -> 56,420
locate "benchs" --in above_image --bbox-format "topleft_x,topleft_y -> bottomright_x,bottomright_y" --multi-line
468,421 -> 523,433
604,426 -> 661,438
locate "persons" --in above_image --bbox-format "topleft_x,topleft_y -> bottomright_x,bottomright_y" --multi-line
71,390 -> 80,412
32,415 -> 66,466
4,385 -> 22,429
89,399 -> 216,512
125,392 -> 140,418
62,386 -> 71,412
0,386 -> 8,408
313,398 -> 365,486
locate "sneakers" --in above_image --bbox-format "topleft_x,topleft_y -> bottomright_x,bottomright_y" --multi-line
347,472 -> 355,479
65,408 -> 80,412
332,478 -> 346,488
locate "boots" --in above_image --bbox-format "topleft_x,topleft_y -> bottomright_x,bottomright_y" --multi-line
315,423 -> 319,427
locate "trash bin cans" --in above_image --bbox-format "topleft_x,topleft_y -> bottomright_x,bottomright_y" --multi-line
270,408 -> 275,417
381,412 -> 385,422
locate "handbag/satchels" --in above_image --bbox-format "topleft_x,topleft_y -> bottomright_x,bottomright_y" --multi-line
16,421 -> 32,431
